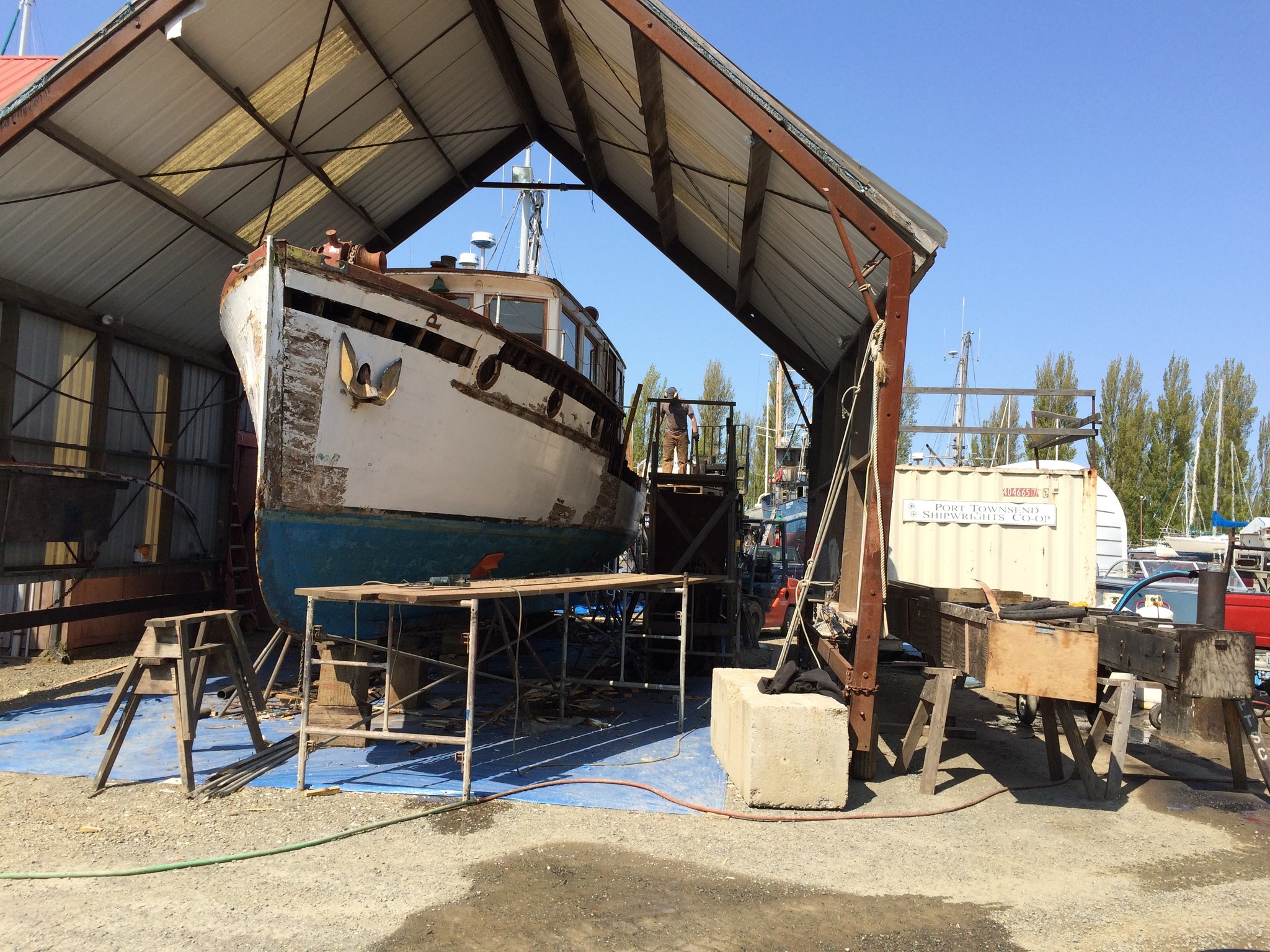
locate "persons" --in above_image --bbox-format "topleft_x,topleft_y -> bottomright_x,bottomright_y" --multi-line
656,387 -> 700,474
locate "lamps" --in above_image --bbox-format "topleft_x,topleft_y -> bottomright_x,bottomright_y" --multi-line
836,335 -> 853,348
510,166 -> 534,190
96,312 -> 125,326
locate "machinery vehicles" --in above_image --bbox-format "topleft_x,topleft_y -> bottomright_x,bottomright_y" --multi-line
729,518 -> 801,641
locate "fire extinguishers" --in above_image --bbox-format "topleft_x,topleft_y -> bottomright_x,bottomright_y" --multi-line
775,526 -> 781,546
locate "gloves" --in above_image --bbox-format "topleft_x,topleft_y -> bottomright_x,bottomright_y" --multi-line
692,432 -> 699,442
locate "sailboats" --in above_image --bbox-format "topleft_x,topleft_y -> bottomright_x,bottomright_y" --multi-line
741,351 -> 814,563
1096,377 -> 1270,650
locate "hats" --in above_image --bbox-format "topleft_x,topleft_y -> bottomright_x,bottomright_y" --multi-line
667,388 -> 676,399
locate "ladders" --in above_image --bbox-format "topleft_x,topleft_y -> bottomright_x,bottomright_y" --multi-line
224,488 -> 260,632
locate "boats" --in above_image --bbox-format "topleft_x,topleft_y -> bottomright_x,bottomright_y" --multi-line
217,147 -> 650,646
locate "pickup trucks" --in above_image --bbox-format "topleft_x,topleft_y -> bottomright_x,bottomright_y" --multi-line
745,545 -> 804,582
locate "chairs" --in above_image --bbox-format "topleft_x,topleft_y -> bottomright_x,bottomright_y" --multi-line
747,551 -> 773,579
